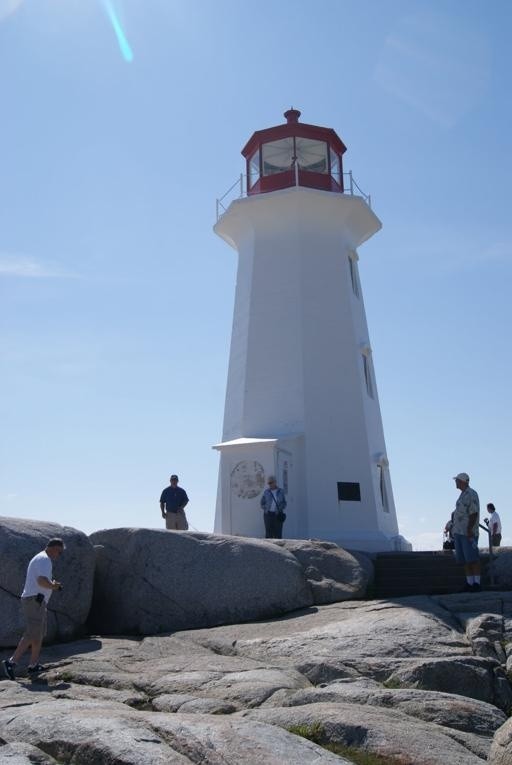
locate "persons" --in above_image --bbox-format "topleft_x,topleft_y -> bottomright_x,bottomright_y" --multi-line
443,511 -> 457,550
260,473 -> 287,539
0,535 -> 67,686
158,473 -> 190,531
483,502 -> 502,546
450,472 -> 484,593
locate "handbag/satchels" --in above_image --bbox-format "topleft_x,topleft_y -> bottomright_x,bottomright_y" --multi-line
279,512 -> 286,523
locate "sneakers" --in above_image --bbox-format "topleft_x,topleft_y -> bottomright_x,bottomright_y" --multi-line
458,582 -> 482,592
1,659 -> 17,681
27,665 -> 49,676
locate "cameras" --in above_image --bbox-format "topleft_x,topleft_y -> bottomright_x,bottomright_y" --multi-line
51,577 -> 65,592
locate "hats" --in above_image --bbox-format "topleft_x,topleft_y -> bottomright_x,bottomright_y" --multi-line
170,475 -> 179,480
453,472 -> 469,483
